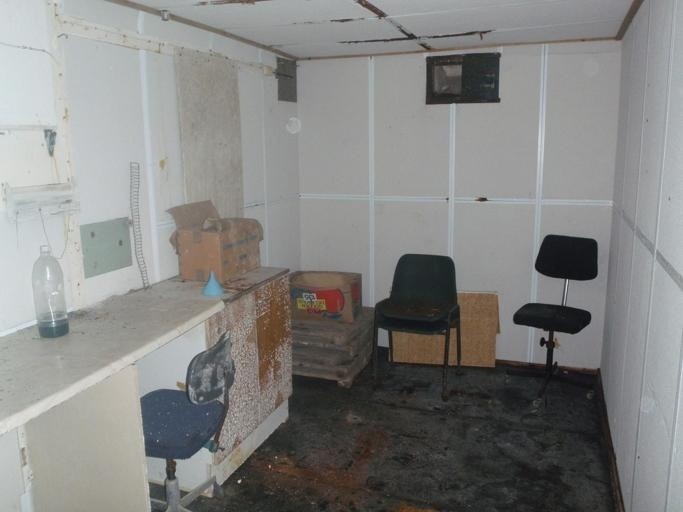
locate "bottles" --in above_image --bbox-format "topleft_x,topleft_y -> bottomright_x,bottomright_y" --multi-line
31,245 -> 70,339
203,272 -> 223,297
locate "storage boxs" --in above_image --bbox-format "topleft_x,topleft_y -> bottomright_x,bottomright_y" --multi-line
288,270 -> 362,325
166,199 -> 262,286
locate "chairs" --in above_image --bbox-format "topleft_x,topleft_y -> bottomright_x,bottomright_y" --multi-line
369,255 -> 461,404
510,233 -> 599,411
139,325 -> 238,512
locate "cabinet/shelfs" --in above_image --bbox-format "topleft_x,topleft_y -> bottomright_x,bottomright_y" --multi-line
0,364 -> 150,512
135,274 -> 293,497
